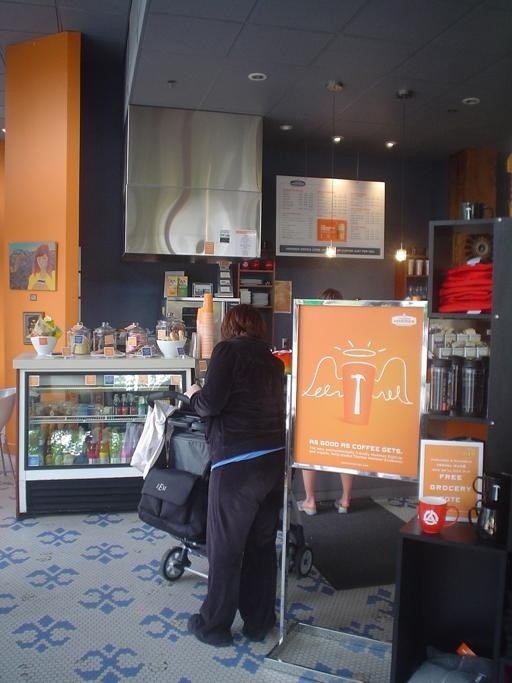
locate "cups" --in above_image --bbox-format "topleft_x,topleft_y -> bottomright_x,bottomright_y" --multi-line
418,495 -> 459,535
341,362 -> 377,425
469,471 -> 511,549
405,246 -> 429,276
189,293 -> 215,360
319,224 -> 346,241
459,201 -> 494,219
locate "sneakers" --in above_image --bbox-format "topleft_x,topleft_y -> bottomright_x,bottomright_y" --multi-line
187,615 -> 232,645
243,614 -> 276,641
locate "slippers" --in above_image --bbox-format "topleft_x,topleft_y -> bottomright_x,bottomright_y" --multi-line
297,501 -> 316,516
334,498 -> 347,513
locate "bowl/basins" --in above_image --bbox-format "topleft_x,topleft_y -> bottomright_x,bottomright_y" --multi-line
30,336 -> 57,354
240,278 -> 269,305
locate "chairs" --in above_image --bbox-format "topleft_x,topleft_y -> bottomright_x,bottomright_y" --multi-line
0,387 -> 17,481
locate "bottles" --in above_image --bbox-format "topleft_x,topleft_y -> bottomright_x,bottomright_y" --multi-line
67,312 -> 187,357
29,392 -> 145,465
407,281 -> 428,301
427,329 -> 491,416
240,257 -> 272,269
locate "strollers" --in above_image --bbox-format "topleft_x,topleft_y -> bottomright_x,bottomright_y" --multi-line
138,387 -> 316,584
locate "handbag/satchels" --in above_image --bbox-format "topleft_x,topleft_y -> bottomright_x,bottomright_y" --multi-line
408,646 -> 493,683
138,465 -> 207,545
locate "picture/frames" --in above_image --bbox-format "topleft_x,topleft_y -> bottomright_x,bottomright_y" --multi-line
22,312 -> 45,345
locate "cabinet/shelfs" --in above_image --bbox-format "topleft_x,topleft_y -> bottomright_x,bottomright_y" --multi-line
421,222 -> 512,476
233,263 -> 275,345
388,511 -> 508,683
18,368 -> 194,521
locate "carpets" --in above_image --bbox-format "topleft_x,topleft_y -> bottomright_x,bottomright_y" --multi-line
289,496 -> 407,591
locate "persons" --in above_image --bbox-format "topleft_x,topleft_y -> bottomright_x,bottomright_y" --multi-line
184,303 -> 285,646
297,469 -> 353,516
319,286 -> 344,299
